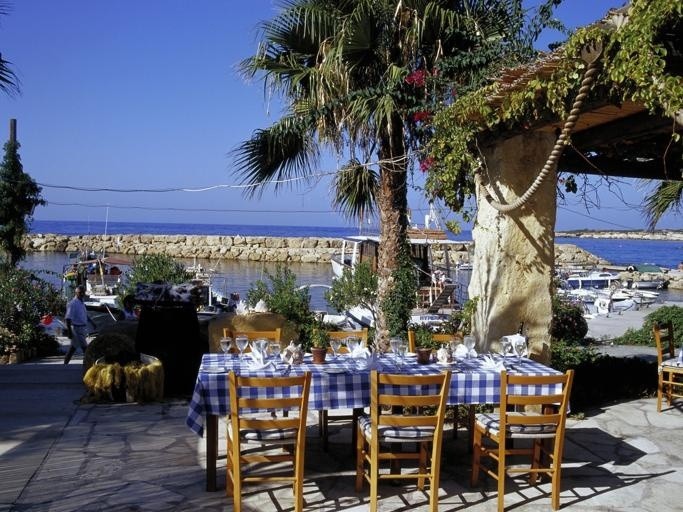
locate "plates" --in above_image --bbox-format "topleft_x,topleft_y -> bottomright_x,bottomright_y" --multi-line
436,361 -> 457,365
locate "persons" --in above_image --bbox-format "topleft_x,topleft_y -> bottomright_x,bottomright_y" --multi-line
64,286 -> 96,365
118,296 -> 135,320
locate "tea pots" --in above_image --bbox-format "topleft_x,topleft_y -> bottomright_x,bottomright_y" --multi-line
437,343 -> 452,363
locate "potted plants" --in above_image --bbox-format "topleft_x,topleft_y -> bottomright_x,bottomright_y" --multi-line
414,328 -> 434,365
309,327 -> 330,364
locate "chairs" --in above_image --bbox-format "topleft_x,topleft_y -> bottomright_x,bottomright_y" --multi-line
472,368 -> 574,512
354,369 -> 452,511
224,329 -> 281,448
223,371 -> 314,511
310,327 -> 369,437
653,320 -> 683,413
405,330 -> 464,441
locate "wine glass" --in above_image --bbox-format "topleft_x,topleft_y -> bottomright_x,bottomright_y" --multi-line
220,336 -> 282,365
498,337 -> 525,368
329,336 -> 358,364
463,334 -> 476,362
389,337 -> 409,370
